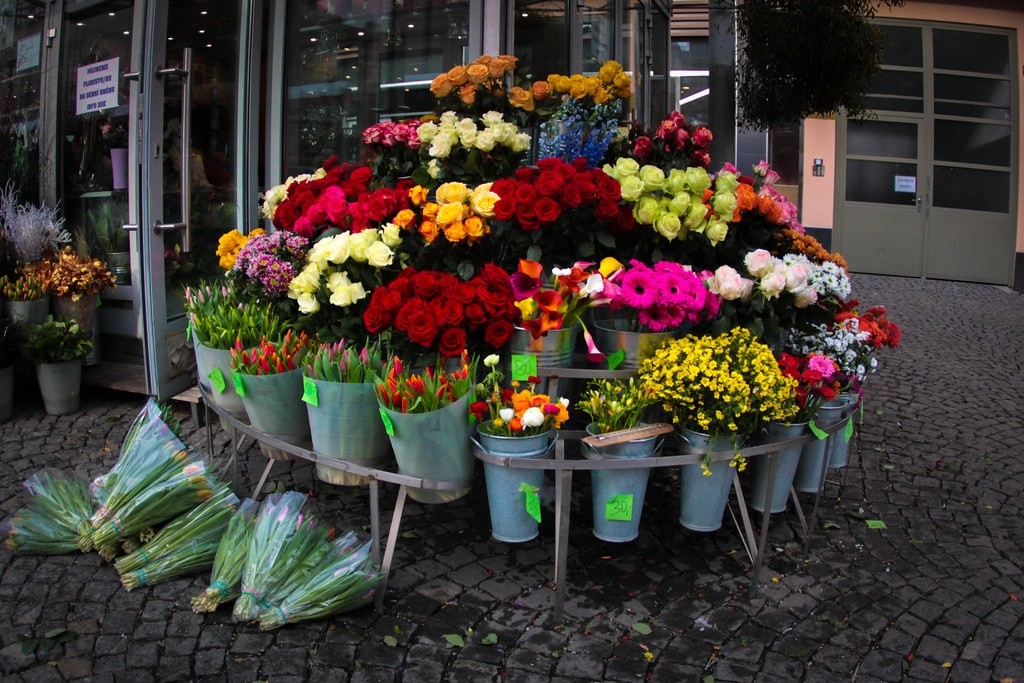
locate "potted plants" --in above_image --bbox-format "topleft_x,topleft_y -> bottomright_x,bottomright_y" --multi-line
103,125 -> 128,189
0,179 -> 117,420
88,196 -> 131,285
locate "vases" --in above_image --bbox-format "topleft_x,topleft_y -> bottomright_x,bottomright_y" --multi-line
594,319 -> 679,372
7,297 -> 48,324
506,321 -> 579,397
191,330 -> 858,543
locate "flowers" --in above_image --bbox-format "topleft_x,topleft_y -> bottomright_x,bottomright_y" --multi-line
184,55 -> 901,475
0,275 -> 50,301
0,397 -> 386,630
164,244 -> 195,284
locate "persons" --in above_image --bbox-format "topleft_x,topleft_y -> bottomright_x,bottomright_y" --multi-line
165,116 -> 213,188
199,85 -> 228,154
